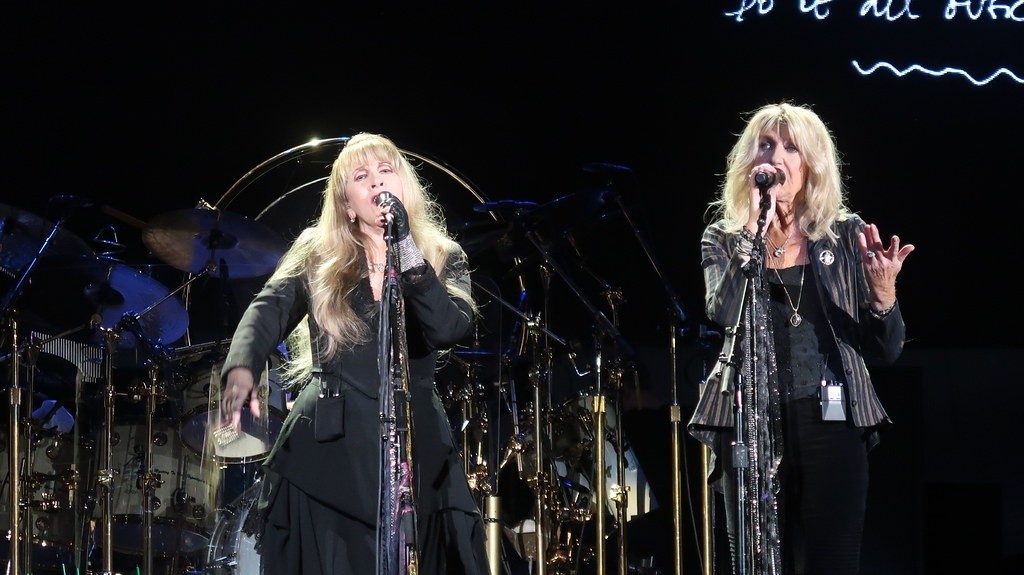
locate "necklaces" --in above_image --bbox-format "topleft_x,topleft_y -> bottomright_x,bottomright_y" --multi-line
762,234 -> 813,328
366,257 -> 387,273
765,226 -> 798,259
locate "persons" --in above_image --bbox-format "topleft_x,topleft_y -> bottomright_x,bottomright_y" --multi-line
222,132 -> 474,575
699,102 -> 915,574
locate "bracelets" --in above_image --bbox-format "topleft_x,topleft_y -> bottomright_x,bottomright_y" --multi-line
393,234 -> 425,272
871,302 -> 894,317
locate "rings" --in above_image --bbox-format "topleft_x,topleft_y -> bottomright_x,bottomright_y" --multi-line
866,251 -> 877,260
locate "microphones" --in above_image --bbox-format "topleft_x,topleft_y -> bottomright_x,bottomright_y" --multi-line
755,168 -> 787,187
582,162 -> 633,174
375,191 -> 394,224
485,200 -> 538,211
